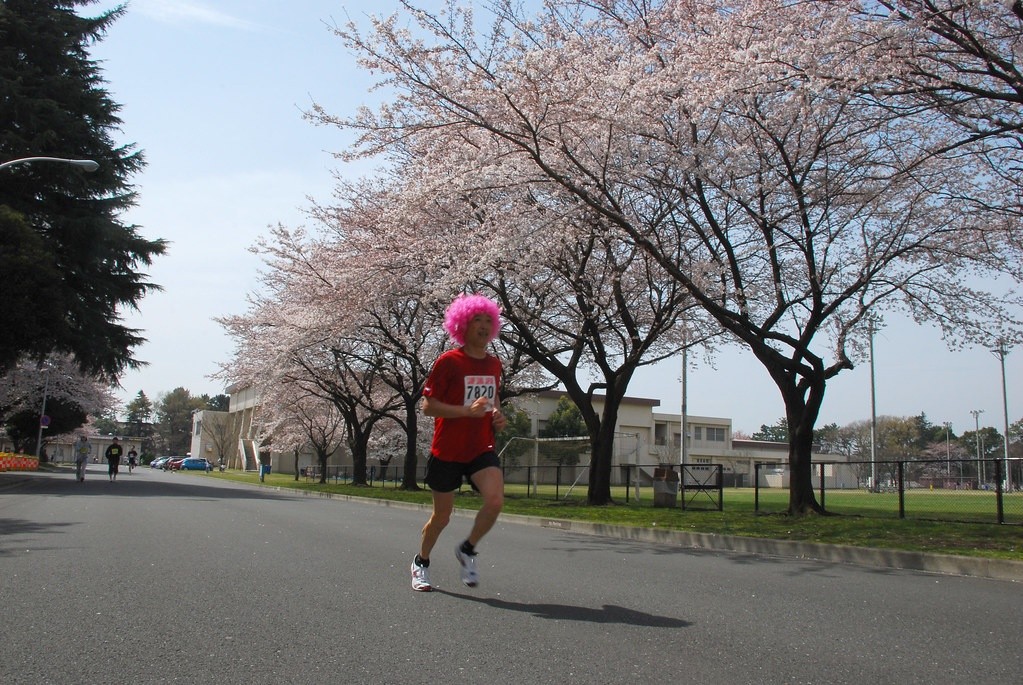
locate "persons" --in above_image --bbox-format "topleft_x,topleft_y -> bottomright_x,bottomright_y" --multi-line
128,445 -> 137,474
410,296 -> 509,591
105,437 -> 122,482
76,435 -> 91,481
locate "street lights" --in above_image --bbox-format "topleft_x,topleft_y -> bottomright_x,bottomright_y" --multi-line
35,367 -> 51,458
990,340 -> 1015,493
976,433 -> 990,489
942,421 -> 953,489
970,410 -> 986,489
860,318 -> 882,492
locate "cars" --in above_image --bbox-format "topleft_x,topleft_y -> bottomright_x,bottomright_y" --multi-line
180,458 -> 213,471
150,456 -> 210,471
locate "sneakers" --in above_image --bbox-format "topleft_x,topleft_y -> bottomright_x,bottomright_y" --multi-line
411,553 -> 431,591
454,544 -> 478,588
